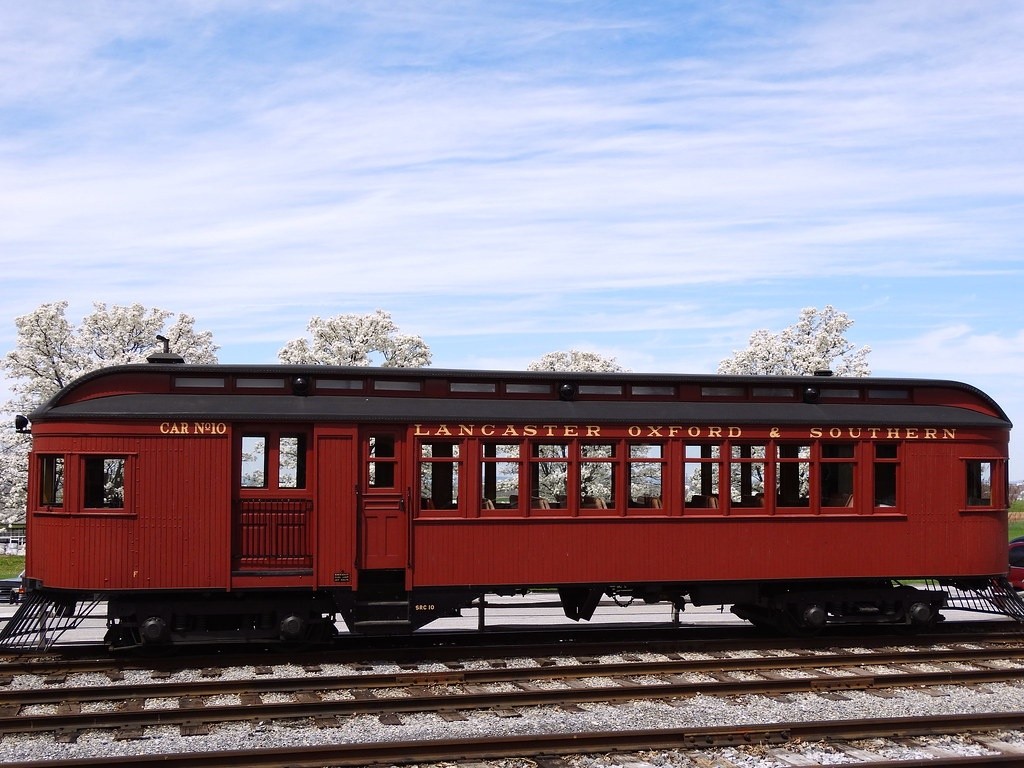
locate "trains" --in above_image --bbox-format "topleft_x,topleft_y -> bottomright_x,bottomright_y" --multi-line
0,336 -> 1024,649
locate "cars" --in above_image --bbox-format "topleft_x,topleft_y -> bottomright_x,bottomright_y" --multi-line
990,536 -> 1024,594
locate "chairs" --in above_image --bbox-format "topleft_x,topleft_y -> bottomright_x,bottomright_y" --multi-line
426,493 -> 855,510
